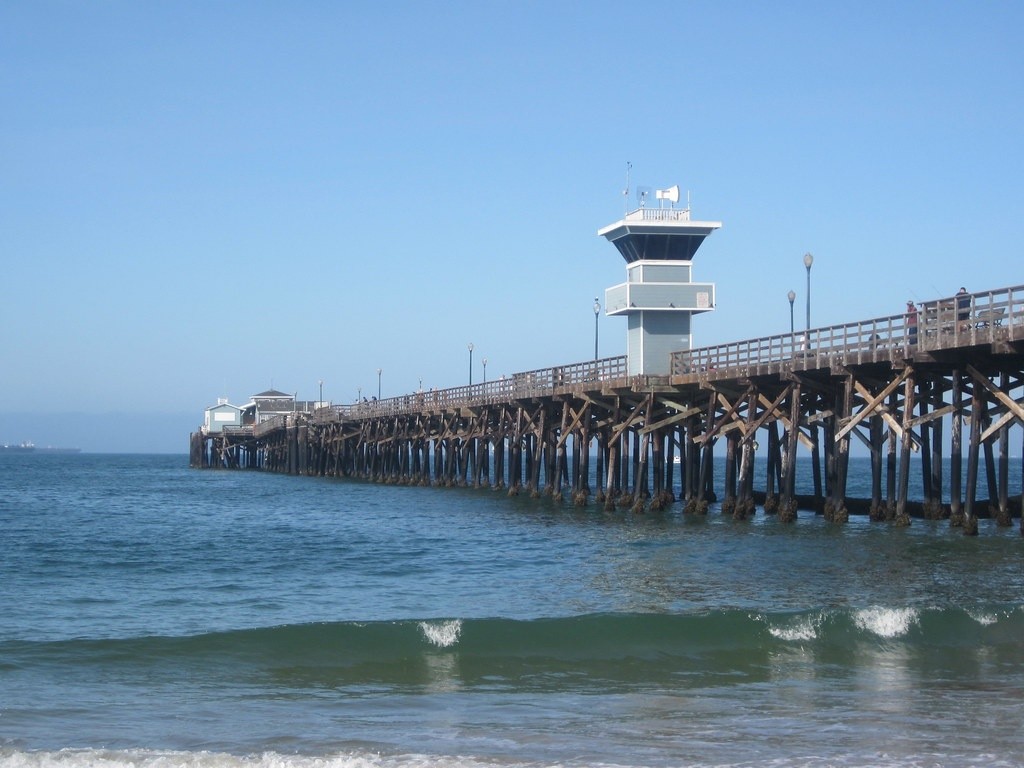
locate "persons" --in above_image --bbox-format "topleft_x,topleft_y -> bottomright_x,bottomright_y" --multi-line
372,396 -> 377,400
363,396 -> 369,405
355,398 -> 361,411
954,287 -> 971,331
906,300 -> 918,345
393,386 -> 439,409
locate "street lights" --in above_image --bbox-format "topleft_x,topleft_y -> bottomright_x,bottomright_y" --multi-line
357,386 -> 361,410
593,298 -> 601,381
481,357 -> 487,398
788,291 -> 795,358
418,377 -> 423,408
804,253 -> 813,356
293,392 -> 297,418
468,342 -> 474,400
377,368 -> 382,408
318,379 -> 324,419
